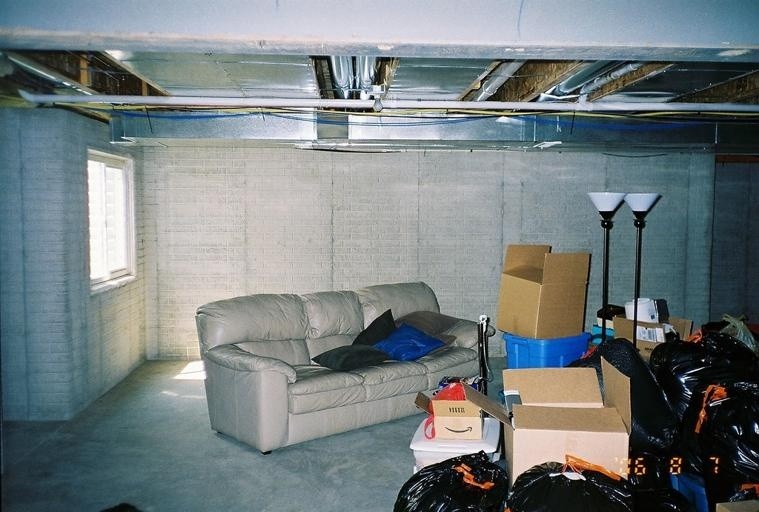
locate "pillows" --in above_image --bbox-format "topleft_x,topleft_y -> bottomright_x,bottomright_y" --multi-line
311,308 -> 463,372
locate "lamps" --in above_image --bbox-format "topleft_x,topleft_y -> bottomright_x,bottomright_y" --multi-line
586,192 -> 660,349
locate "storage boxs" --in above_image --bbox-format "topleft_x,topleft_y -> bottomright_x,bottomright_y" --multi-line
407,417 -> 503,482
501,333 -> 591,368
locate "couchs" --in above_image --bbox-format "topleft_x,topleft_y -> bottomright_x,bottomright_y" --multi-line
195,281 -> 495,456
